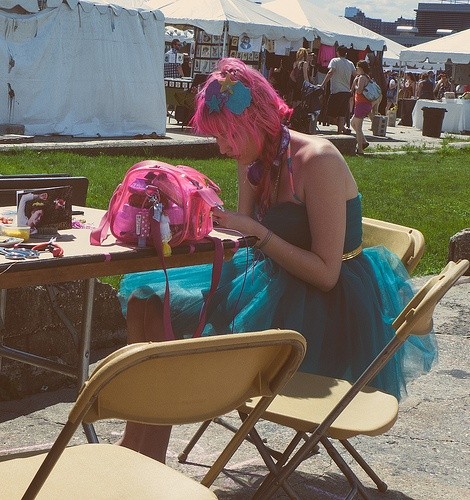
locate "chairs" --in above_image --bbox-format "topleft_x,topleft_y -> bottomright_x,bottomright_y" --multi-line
0,218 -> 470,500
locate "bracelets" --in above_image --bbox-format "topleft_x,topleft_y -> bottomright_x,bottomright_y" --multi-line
254,229 -> 275,250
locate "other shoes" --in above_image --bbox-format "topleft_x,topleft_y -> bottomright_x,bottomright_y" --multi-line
168,113 -> 173,117
344,129 -> 351,135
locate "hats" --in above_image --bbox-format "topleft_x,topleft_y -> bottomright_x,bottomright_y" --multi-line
428,71 -> 434,74
307,49 -> 315,55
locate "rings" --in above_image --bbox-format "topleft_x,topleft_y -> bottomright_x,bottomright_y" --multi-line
216,217 -> 220,225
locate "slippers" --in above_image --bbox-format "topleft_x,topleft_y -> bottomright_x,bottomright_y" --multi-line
357,148 -> 364,155
363,142 -> 369,150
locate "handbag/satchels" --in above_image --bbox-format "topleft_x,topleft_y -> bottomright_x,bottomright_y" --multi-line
90,160 -> 225,249
386,87 -> 397,97
356,74 -> 382,101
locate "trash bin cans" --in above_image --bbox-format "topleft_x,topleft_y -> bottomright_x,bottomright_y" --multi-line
421,106 -> 448,138
400,100 -> 417,127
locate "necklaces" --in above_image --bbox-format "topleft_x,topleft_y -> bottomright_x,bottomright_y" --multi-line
268,126 -> 287,200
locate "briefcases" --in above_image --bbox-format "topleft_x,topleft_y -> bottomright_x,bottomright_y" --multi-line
372,113 -> 387,137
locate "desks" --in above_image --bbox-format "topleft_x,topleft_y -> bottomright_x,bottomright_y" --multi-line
0,205 -> 257,460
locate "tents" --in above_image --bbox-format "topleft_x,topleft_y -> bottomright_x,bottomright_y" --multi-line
401,28 -> 470,64
111,0 -> 384,52
382,38 -> 447,72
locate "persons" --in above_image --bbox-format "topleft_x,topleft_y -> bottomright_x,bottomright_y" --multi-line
17,193 -> 48,234
349,60 -> 372,155
165,38 -> 184,77
397,71 -> 454,118
289,48 -> 312,134
109,58 -> 440,466
385,71 -> 399,116
316,45 -> 356,134
